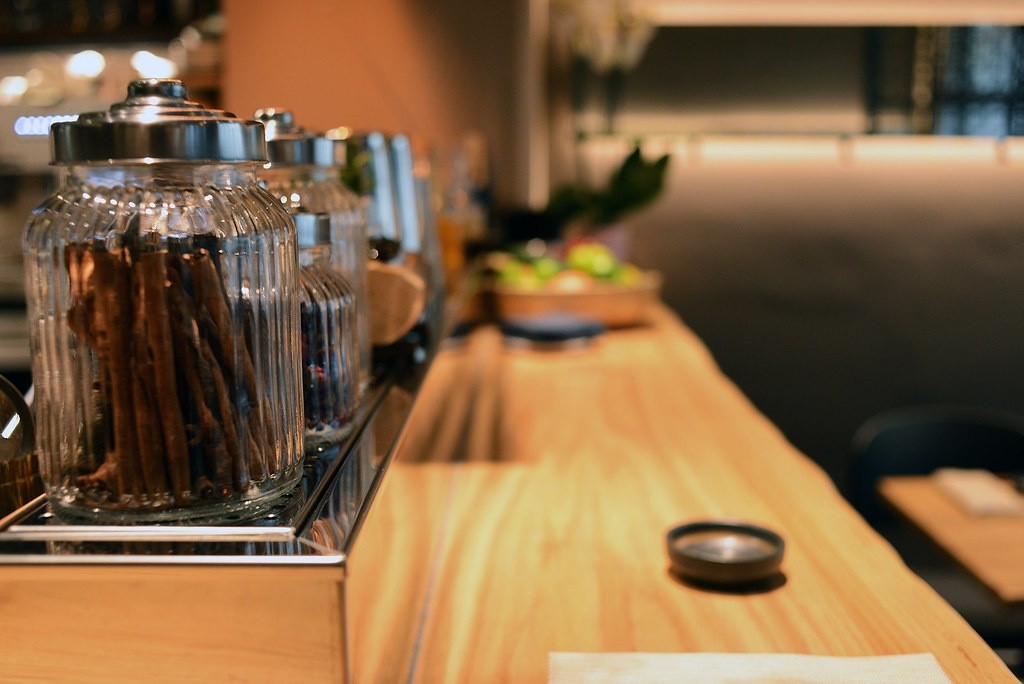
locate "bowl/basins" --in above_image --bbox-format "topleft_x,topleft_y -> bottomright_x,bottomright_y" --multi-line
487,271 -> 660,329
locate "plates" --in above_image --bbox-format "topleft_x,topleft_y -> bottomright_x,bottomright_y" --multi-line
667,523 -> 784,581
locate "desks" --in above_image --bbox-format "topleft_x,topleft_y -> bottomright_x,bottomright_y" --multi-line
402,280 -> 1024,684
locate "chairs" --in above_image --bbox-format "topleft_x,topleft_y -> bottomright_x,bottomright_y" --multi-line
840,406 -> 1024,646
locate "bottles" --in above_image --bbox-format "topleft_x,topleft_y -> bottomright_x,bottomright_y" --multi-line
19,77 -> 415,524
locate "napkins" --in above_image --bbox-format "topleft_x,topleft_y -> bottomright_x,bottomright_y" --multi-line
549,650 -> 952,683
